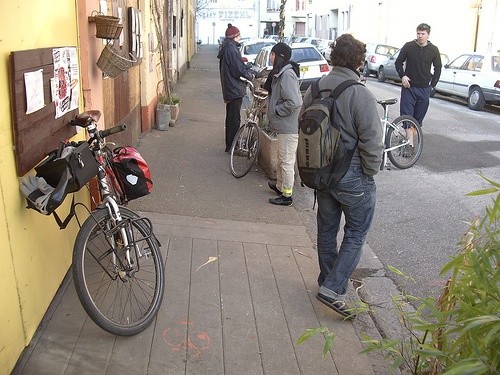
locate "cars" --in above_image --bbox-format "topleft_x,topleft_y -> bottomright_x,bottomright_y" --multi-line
376,47 -> 450,87
430,52 -> 500,111
216,33 -> 401,93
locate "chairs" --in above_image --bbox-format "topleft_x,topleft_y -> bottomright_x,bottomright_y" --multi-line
293,53 -> 304,60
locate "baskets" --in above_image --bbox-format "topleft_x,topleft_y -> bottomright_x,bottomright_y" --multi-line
96,11 -> 123,40
97,40 -> 137,78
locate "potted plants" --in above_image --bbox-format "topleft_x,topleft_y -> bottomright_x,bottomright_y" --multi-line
161,96 -> 181,127
256,118 -> 299,180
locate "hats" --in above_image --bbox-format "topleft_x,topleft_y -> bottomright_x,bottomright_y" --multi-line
271,43 -> 291,61
226,26 -> 240,39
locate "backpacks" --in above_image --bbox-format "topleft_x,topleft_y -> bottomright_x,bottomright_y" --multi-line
297,78 -> 366,192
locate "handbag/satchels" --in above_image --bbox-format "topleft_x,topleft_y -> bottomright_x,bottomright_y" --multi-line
107,146 -> 154,201
34,140 -> 98,193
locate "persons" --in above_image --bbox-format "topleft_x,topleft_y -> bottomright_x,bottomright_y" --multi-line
218,25 -> 255,157
263,43 -> 303,206
297,33 -> 384,321
395,23 -> 442,157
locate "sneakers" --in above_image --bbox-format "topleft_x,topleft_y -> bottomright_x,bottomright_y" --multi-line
316,292 -> 357,319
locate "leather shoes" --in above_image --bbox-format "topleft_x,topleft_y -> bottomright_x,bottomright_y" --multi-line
268,182 -> 282,195
269,195 -> 293,206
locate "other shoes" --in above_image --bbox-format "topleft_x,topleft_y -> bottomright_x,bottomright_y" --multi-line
225,148 -> 247,157
399,145 -> 415,157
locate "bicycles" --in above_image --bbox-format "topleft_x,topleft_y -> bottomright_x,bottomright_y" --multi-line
376,97 -> 424,171
31,109 -> 167,339
227,75 -> 269,179
